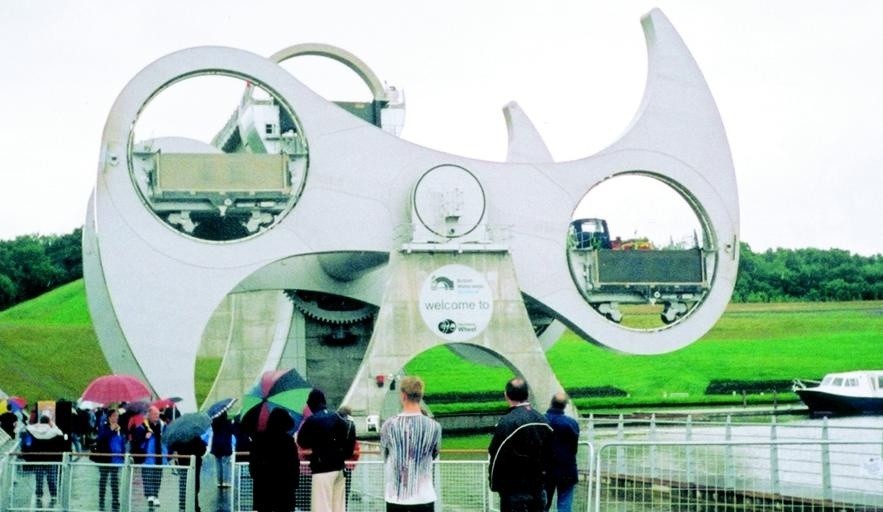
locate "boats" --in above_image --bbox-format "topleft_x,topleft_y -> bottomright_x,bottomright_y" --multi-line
792,369 -> 883,411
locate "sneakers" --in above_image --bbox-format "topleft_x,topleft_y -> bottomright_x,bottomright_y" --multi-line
148,496 -> 160,506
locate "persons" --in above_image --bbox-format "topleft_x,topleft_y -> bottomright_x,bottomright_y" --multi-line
487,377 -> 579,512
378,376 -> 442,512
0,389 -> 360,512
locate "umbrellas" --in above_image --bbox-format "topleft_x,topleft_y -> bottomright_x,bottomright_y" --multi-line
240,367 -> 314,436
